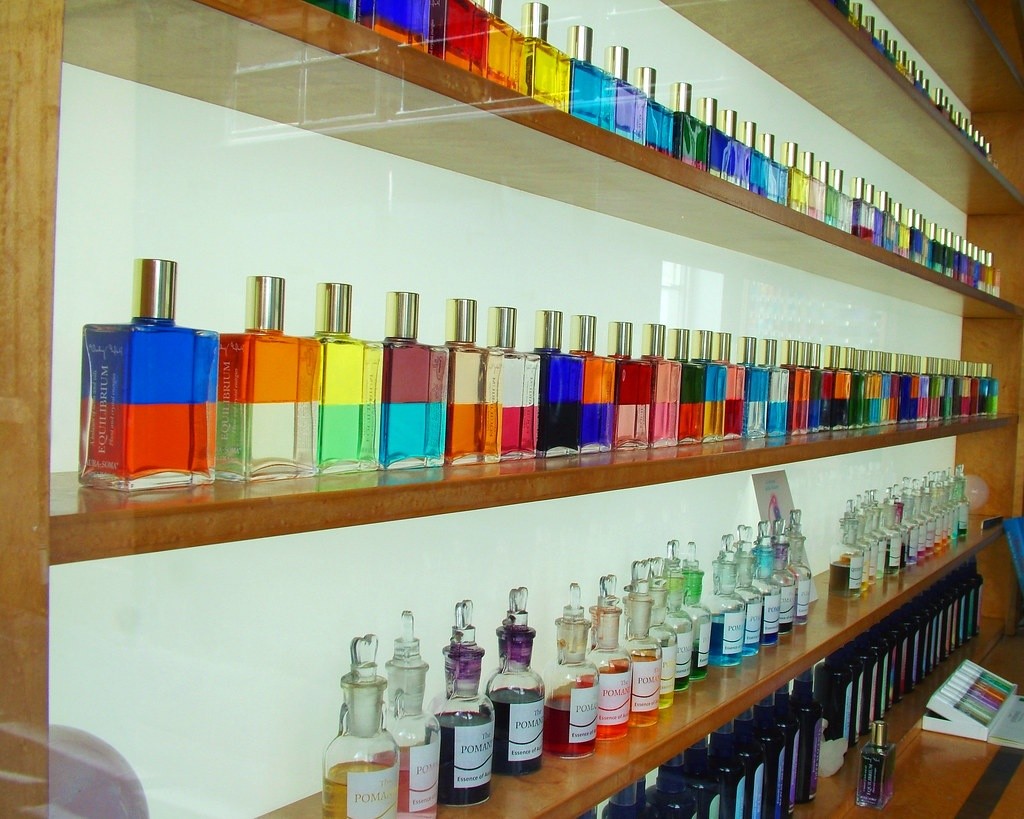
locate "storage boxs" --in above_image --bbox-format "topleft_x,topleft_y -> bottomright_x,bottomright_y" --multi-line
922,660 -> 1017,742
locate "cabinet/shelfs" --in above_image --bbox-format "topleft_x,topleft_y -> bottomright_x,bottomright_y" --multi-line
0,0 -> 1024,819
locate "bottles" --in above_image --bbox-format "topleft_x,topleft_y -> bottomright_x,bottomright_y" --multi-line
81,0 -> 999,819
